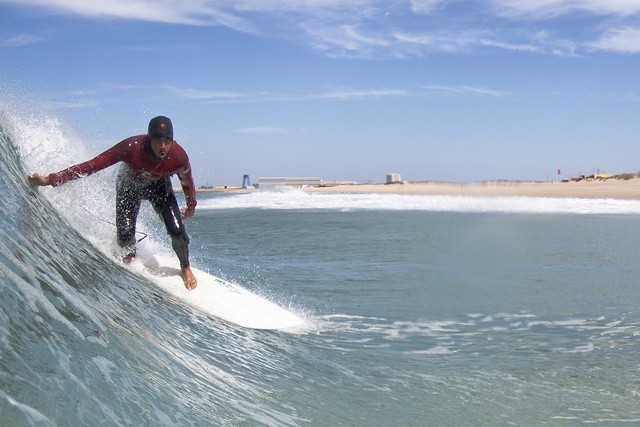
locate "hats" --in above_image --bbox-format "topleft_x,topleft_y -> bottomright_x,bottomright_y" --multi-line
148,116 -> 173,140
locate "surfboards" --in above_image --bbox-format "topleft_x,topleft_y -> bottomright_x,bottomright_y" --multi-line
113,252 -> 306,330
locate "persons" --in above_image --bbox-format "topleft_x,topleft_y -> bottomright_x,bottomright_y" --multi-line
29,116 -> 197,290
